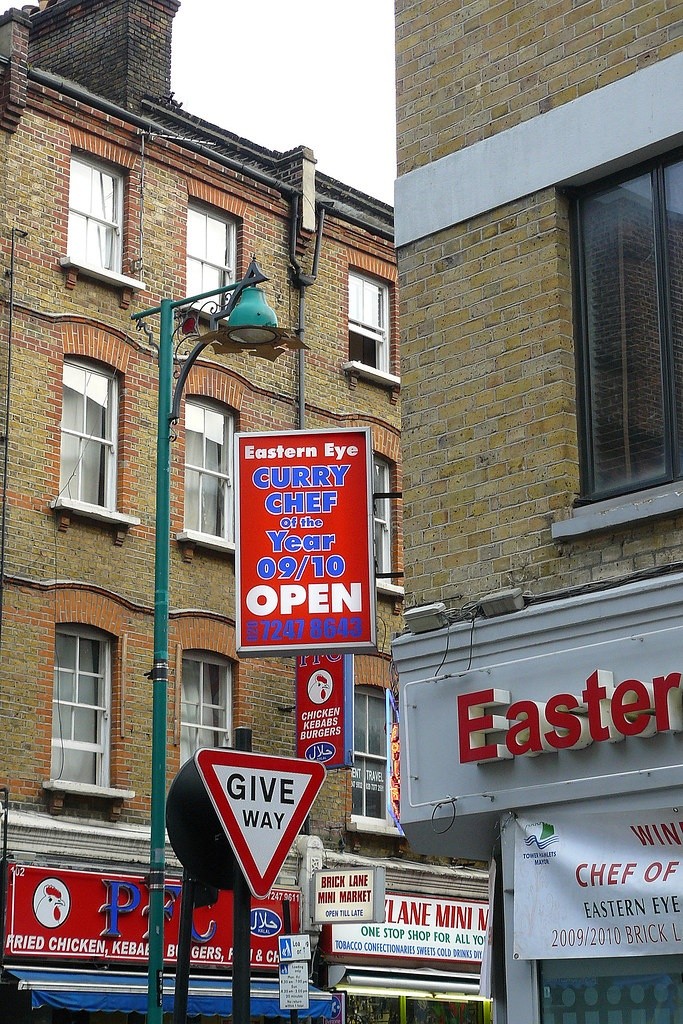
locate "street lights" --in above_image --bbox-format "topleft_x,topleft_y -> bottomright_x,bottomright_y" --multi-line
130,252 -> 311,1024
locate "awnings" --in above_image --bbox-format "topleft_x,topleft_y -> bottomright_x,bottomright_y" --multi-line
1,964 -> 332,1020
